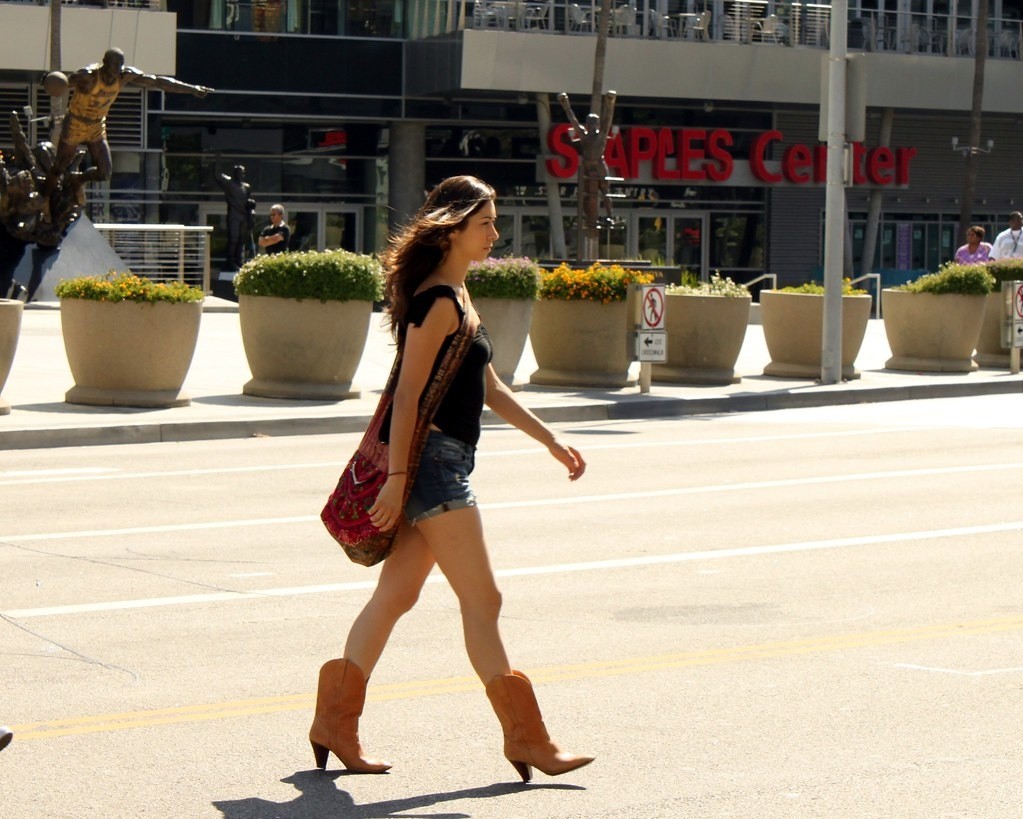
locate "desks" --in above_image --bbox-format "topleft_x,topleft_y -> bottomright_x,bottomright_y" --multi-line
672,13 -> 697,31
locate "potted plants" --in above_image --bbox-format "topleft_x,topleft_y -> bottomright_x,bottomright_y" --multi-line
882,261 -> 996,372
325,215 -> 345,244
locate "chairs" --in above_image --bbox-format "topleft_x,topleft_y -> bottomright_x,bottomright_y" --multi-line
570,4 -> 636,36
724,13 -> 781,43
473,1 -> 551,30
872,15 -> 1019,59
649,9 -> 711,41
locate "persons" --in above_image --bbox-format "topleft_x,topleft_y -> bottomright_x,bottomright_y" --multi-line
989,211 -> 1023,261
213,150 -> 256,272
259,204 -> 290,255
43,48 -> 216,211
955,225 -> 994,265
309,175 -> 595,781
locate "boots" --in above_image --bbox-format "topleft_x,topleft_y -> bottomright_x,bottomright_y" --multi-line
309,658 -> 393,774
487,670 -> 596,781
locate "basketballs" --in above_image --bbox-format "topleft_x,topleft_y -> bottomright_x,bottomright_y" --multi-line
44,70 -> 69,96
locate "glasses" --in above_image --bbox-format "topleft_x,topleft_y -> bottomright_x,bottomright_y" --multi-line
269,212 -> 278,216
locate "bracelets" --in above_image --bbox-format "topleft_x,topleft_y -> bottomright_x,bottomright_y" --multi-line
386,471 -> 407,478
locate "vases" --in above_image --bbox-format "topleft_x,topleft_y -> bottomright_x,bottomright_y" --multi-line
760,290 -> 872,380
470,297 -> 532,389
639,295 -> 752,385
61,299 -> 203,407
238,295 -> 373,400
972,292 -> 1023,370
0,298 -> 24,416
529,298 -> 639,385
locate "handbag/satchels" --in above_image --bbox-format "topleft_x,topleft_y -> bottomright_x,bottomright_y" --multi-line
319,440 -> 418,568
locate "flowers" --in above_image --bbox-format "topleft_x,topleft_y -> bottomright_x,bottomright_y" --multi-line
982,258 -> 1023,292
232,247 -> 387,304
537,261 -> 655,305
465,253 -> 540,301
664,268 -> 752,297
771,277 -> 868,295
55,269 -> 205,310
